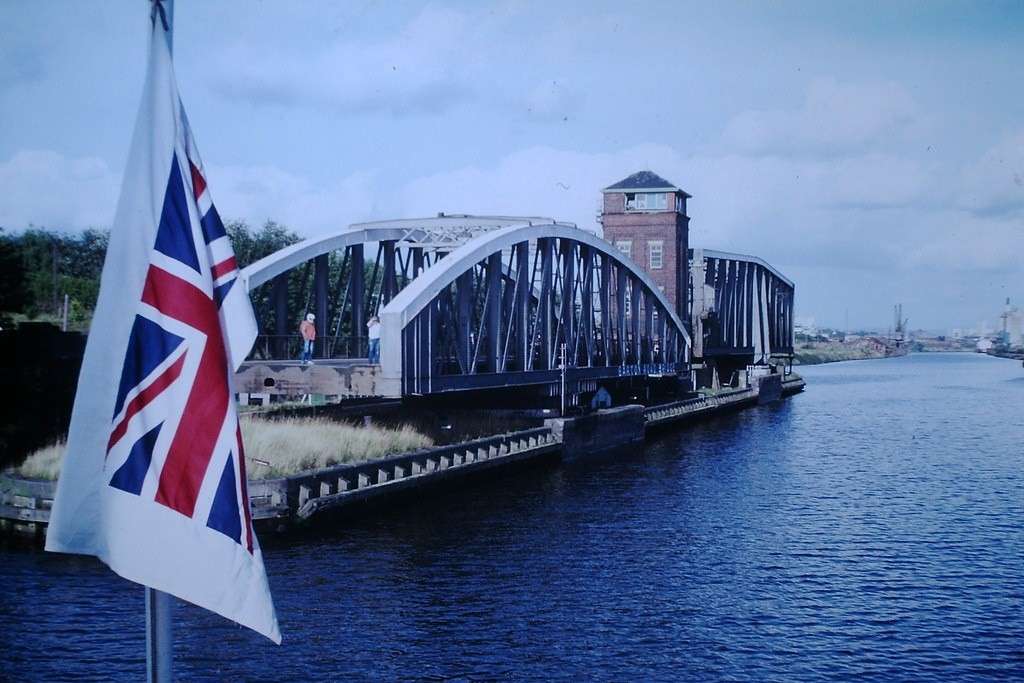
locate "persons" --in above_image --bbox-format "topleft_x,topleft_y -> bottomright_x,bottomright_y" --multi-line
366,316 -> 380,364
300,313 -> 316,365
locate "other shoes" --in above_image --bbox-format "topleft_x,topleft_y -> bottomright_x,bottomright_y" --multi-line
374,360 -> 380,364
301,360 -> 305,364
367,361 -> 372,364
307,360 -> 315,365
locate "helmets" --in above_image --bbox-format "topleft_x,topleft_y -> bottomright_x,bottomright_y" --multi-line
306,313 -> 315,324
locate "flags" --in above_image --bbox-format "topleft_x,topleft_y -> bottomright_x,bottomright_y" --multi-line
43,3 -> 283,647
